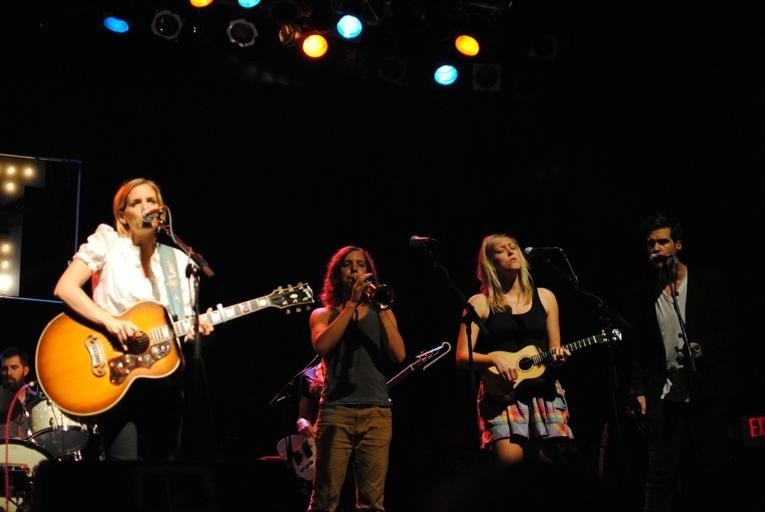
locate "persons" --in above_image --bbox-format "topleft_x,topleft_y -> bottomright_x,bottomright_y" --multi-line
298,363 -> 356,512
1,345 -> 35,424
305,244 -> 408,511
52,177 -> 216,458
626,208 -> 740,511
450,233 -> 573,468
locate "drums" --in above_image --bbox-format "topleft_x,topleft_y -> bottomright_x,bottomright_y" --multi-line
0,437 -> 56,512
24,399 -> 92,455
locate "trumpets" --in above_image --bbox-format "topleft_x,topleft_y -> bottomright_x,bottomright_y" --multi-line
353,274 -> 395,311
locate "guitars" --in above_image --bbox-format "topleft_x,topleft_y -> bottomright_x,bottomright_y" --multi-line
276,341 -> 451,482
34,282 -> 314,417
478,327 -> 623,398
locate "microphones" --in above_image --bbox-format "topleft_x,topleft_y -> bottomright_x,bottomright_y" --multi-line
523,246 -> 563,259
142,206 -> 165,224
408,235 -> 441,248
648,253 -> 673,266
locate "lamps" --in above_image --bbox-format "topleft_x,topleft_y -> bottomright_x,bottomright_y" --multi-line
227,15 -> 264,52
429,0 -> 511,89
269,1 -> 380,67
150,7 -> 190,45
